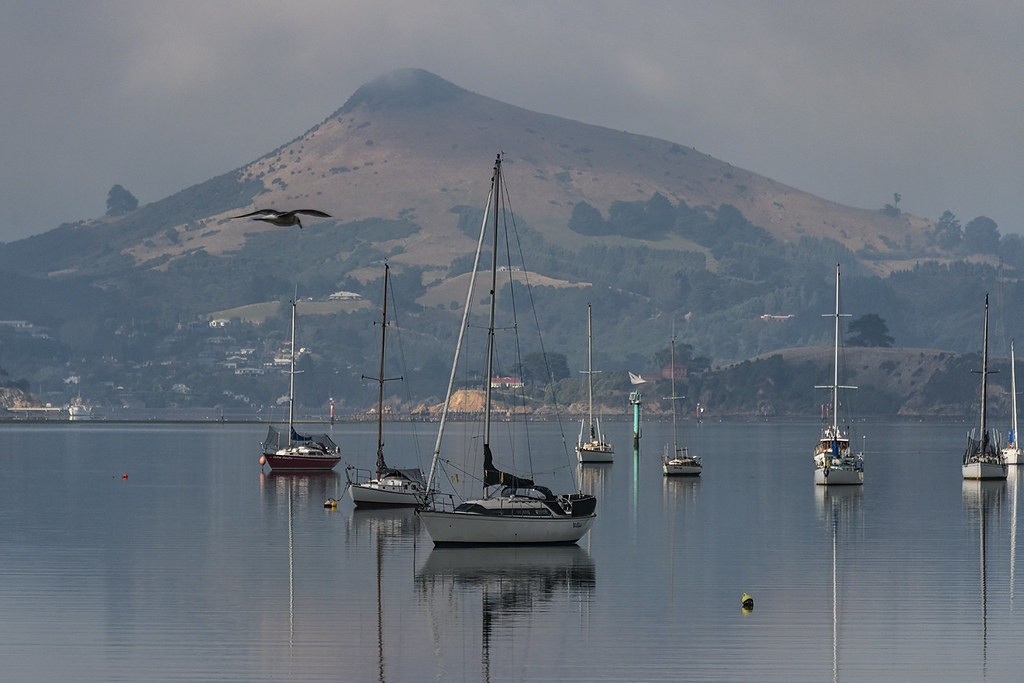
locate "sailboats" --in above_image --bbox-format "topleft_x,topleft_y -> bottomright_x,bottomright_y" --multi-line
993,341 -> 1024,464
408,153 -> 600,549
811,260 -> 865,486
658,321 -> 705,476
961,291 -> 1011,481
573,301 -> 616,463
344,261 -> 441,506
262,283 -> 342,471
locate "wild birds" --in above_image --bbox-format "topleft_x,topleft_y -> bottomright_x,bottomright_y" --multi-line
229,209 -> 332,229
628,372 -> 647,384
